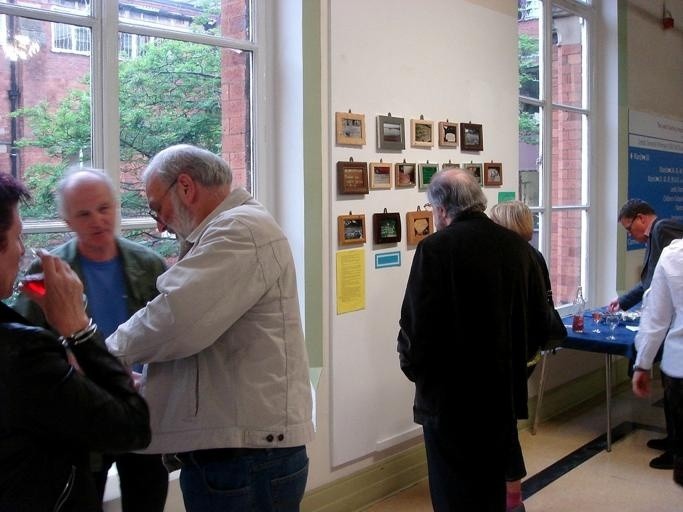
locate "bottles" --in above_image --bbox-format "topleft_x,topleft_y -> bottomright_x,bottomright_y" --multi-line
571,285 -> 585,334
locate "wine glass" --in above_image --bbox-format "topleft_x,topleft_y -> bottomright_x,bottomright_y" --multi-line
590,306 -> 621,342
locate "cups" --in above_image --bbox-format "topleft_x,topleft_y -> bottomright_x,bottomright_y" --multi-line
11,247 -> 88,316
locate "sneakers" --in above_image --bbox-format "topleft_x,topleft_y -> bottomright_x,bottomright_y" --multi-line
647,438 -> 668,451
650,450 -> 675,469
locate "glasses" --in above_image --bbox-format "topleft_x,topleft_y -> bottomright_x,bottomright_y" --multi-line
146,178 -> 178,224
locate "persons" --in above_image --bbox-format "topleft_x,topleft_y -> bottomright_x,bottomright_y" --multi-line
9,169 -> 167,512
103,143 -> 315,512
608,199 -> 682,471
626,231 -> 682,488
0,174 -> 152,512
488,196 -> 553,512
396,166 -> 545,512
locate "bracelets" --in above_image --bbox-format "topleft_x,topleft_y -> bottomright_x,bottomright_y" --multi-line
59,319 -> 99,349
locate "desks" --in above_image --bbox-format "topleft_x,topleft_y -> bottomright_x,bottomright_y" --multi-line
532,298 -> 671,452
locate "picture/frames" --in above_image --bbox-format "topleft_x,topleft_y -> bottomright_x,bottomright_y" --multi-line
370,162 -> 392,188
337,161 -> 368,194
438,122 -> 459,147
335,112 -> 366,145
459,123 -> 483,151
373,213 -> 401,244
483,160 -> 501,185
463,163 -> 482,187
395,162 -> 416,186
412,119 -> 434,148
375,114 -> 404,150
443,163 -> 460,171
338,215 -> 367,244
406,211 -> 434,246
419,163 -> 439,189
